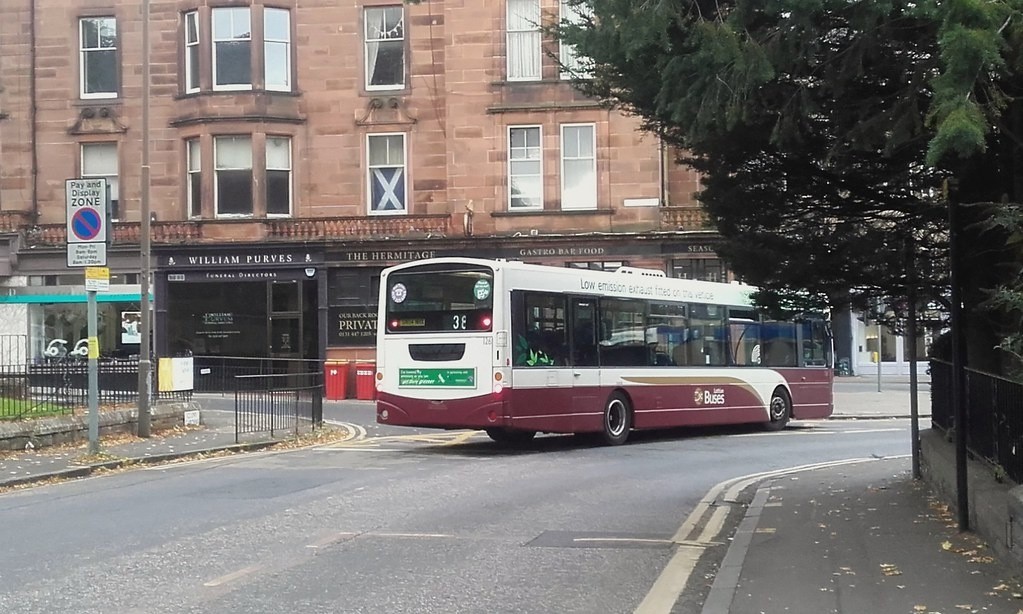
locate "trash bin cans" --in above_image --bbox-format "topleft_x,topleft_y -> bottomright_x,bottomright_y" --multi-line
354,360 -> 377,400
323,360 -> 349,401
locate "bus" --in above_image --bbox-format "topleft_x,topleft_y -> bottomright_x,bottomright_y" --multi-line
375,256 -> 835,449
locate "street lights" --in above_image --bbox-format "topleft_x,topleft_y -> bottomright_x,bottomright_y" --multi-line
850,284 -> 883,393
